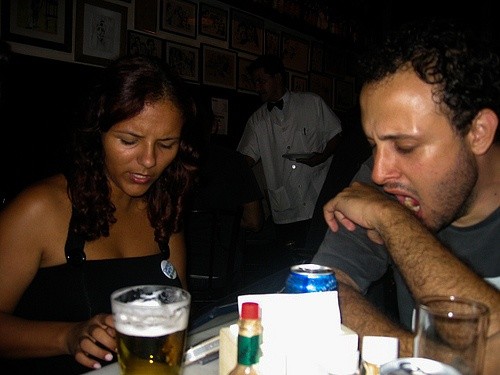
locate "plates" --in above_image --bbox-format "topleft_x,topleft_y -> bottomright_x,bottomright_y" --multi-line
282,153 -> 313,161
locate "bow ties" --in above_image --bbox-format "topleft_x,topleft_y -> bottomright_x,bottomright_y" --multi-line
267,99 -> 285,112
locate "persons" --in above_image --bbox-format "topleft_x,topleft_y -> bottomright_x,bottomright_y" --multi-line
181,55 -> 346,271
307,27 -> 500,375
0,63 -> 198,375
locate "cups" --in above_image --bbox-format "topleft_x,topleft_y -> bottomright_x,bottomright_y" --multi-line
110,285 -> 191,375
410,293 -> 488,375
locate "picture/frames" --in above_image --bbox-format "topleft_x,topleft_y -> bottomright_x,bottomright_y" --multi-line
1,1 -> 319,99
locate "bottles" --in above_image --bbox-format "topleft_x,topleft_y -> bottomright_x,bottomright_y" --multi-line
226,302 -> 264,375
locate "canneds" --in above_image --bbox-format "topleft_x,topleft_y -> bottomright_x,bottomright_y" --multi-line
284,262 -> 337,294
